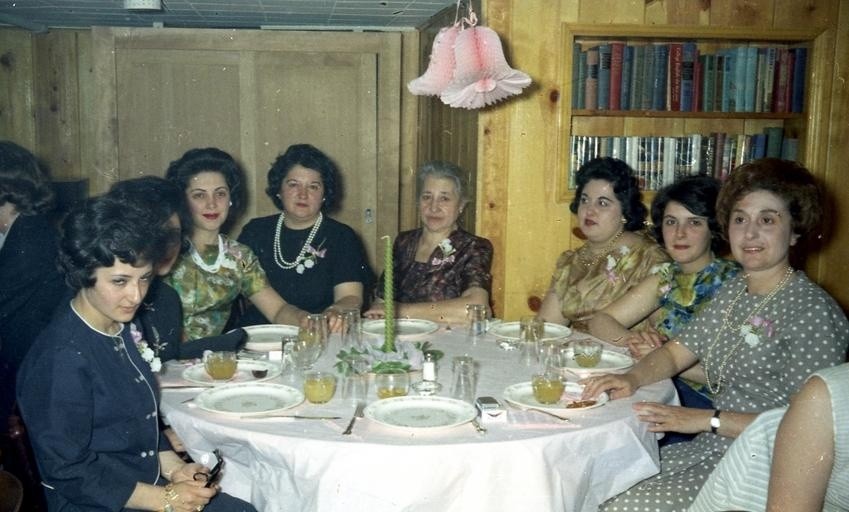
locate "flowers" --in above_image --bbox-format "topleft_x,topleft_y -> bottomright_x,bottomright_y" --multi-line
739,313 -> 762,350
603,244 -> 631,287
657,266 -> 673,298
428,238 -> 456,274
129,322 -> 169,375
295,236 -> 328,275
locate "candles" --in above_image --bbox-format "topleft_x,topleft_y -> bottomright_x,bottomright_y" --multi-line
379,235 -> 395,352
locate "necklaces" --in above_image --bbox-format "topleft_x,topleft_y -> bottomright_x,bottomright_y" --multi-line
580,227 -> 624,266
274,212 -> 323,270
704,266 -> 794,395
187,234 -> 225,274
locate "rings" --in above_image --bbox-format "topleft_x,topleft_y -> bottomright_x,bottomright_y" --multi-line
195,504 -> 203,512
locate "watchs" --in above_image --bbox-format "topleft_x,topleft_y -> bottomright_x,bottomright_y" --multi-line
709,409 -> 721,434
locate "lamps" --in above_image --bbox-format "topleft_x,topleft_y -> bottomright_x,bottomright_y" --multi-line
405,1 -> 533,112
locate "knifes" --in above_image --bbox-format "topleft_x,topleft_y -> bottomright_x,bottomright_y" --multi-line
239,414 -> 344,422
505,399 -> 572,424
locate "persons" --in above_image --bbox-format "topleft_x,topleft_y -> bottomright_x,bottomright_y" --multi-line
574,155 -> 849,511
109,174 -> 192,463
162,147 -> 316,343
587,174 -> 742,445
14,195 -> 257,512
1,140 -> 72,423
534,156 -> 672,333
362,159 -> 495,325
224,143 -> 375,335
683,363 -> 848,511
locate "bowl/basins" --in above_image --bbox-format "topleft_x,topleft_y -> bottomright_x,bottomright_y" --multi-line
192,382 -> 306,417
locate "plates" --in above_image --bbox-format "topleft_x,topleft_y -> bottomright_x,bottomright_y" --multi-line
503,382 -> 610,411
483,320 -> 572,341
227,323 -> 300,353
359,319 -> 440,337
182,360 -> 282,387
364,395 -> 480,432
544,348 -> 635,373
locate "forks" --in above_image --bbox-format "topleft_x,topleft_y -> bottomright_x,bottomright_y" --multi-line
343,403 -> 366,435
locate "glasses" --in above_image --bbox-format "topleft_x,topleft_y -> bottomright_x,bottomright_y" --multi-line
192,447 -> 225,491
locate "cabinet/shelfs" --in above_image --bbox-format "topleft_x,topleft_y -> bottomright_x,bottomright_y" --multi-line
556,20 -> 836,216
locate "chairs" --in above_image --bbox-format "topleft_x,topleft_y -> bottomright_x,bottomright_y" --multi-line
5,412 -> 51,512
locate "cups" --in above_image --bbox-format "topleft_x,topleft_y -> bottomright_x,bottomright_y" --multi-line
448,358 -> 477,403
519,329 -> 546,365
574,340 -> 603,368
520,315 -> 544,340
340,355 -> 370,409
466,304 -> 487,341
339,308 -> 362,350
304,360 -> 337,405
282,335 -> 304,386
413,353 -> 444,395
296,326 -> 323,364
203,347 -> 239,379
533,370 -> 567,404
305,312 -> 330,358
375,369 -> 412,401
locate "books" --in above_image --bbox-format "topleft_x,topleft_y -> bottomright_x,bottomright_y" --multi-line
571,37 -> 808,193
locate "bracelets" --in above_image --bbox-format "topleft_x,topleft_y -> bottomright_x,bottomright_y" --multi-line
163,484 -> 177,512
165,462 -> 185,482
406,301 -> 409,318
